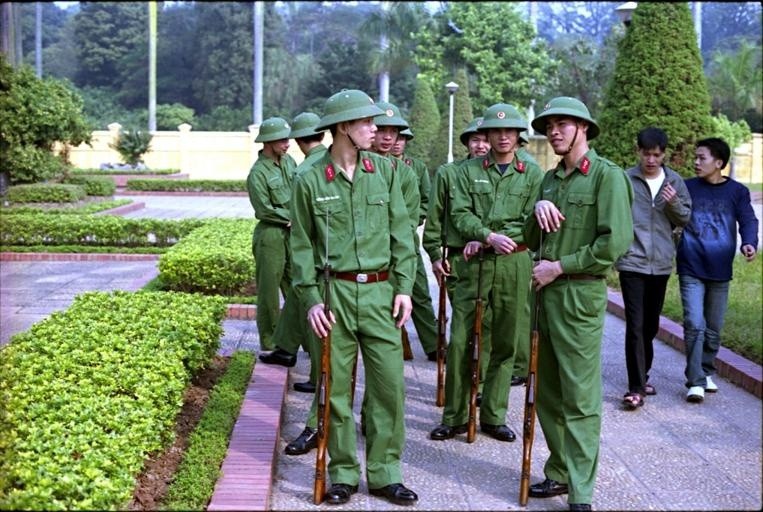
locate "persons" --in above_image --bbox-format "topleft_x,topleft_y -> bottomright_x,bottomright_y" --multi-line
430,103 -> 545,442
675,137 -> 760,402
422,116 -> 494,406
290,89 -> 420,504
390,128 -> 450,365
616,127 -> 692,412
246,117 -> 312,367
520,96 -> 635,512
288,111 -> 330,195
372,101 -> 420,235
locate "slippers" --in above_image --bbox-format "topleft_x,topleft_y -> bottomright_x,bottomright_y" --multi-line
641,384 -> 658,395
621,394 -> 644,406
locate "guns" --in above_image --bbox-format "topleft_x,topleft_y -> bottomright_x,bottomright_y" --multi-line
437,236 -> 447,408
311,205 -> 332,508
402,324 -> 413,361
468,245 -> 482,445
519,215 -> 545,508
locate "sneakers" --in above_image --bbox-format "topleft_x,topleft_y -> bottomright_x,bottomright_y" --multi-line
702,375 -> 719,392
686,386 -> 704,401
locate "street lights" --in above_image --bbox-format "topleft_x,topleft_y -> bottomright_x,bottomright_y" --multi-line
446,81 -> 458,164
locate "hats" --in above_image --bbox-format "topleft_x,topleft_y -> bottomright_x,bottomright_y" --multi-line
459,117 -> 484,145
477,104 -> 528,132
531,96 -> 601,140
399,128 -> 415,140
254,118 -> 292,142
371,103 -> 410,131
314,89 -> 386,130
287,112 -> 328,139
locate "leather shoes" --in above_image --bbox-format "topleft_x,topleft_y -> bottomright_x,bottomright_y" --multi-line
481,421 -> 516,442
455,244 -> 528,256
293,381 -> 317,393
528,478 -> 567,498
258,351 -> 297,367
368,483 -> 417,504
551,274 -> 607,280
472,393 -> 484,407
447,247 -> 468,254
428,348 -> 451,363
429,421 -> 470,441
569,502 -> 591,511
321,270 -> 389,283
324,482 -> 359,504
508,375 -> 531,387
284,428 -> 327,455
